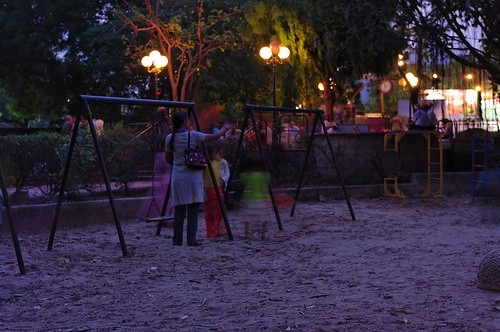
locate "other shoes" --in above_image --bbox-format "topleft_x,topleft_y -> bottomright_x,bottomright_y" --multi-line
187,241 -> 203,246
172,241 -> 182,246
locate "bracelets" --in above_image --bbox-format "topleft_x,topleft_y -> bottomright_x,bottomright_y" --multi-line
224,126 -> 228,131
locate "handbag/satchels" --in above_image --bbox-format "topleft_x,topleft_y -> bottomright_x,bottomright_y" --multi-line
184,131 -> 208,171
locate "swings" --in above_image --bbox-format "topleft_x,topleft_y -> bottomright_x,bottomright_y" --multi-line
147,103 -> 189,223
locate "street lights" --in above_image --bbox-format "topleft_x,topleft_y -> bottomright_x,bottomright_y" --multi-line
399,72 -> 419,130
258,41 -> 291,143
140,49 -> 169,100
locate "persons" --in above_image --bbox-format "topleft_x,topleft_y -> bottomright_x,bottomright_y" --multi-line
387,100 -> 454,139
240,156 -> 272,240
203,145 -> 230,238
212,113 -> 343,151
157,107 -> 172,137
334,103 -> 348,125
60,114 -> 106,139
165,111 -> 237,246
